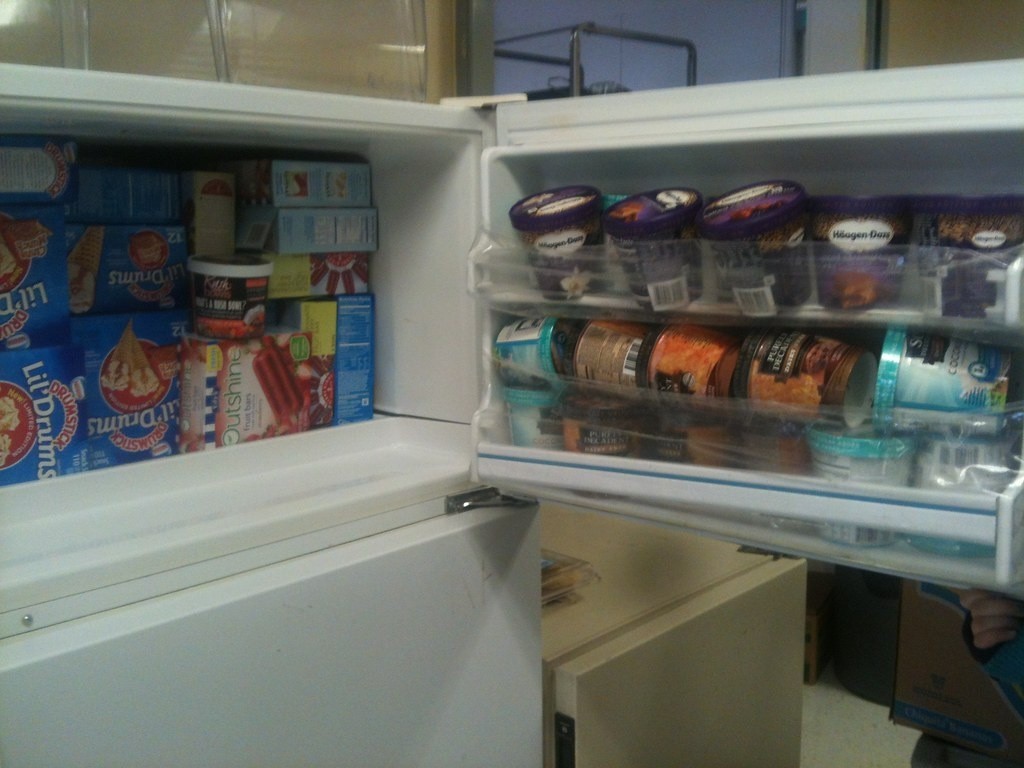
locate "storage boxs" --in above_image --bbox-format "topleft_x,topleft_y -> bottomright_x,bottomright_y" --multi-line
65,222 -> 190,316
804,571 -> 845,685
177,324 -> 312,454
328,293 -> 375,426
0,133 -> 79,207
0,203 -> 77,352
888,577 -> 1024,761
266,299 -> 338,428
73,167 -> 184,225
234,156 -> 370,207
180,168 -> 234,333
68,310 -> 180,469
248,251 -> 368,300
0,340 -> 90,487
237,209 -> 380,255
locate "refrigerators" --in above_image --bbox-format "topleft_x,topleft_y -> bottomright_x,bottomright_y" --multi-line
0,56 -> 1024,768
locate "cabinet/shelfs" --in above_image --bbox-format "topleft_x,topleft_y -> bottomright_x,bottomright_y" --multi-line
538,503 -> 807,767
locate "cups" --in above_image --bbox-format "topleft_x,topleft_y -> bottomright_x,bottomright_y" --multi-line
203,250 -> 270,340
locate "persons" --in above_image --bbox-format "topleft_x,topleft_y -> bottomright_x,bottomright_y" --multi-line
958,587 -> 1024,725
